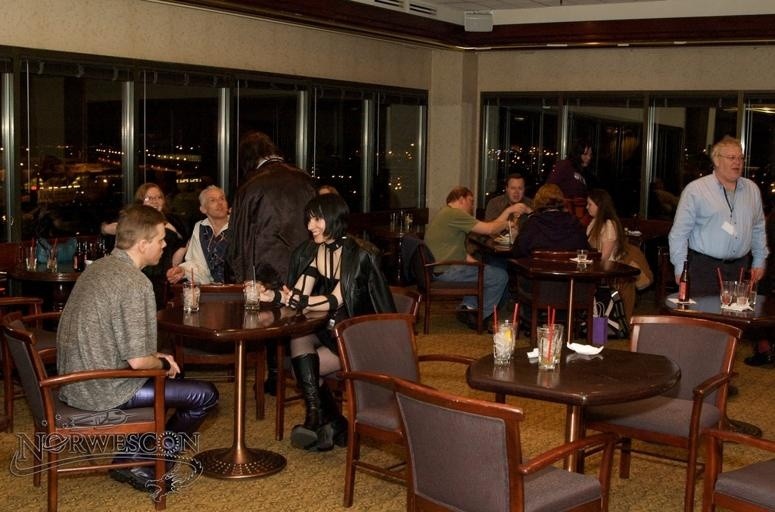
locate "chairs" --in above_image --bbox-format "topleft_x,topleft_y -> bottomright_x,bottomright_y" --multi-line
582,314 -> 742,511
334,311 -> 478,507
387,377 -> 603,511
399,235 -> 484,334
0,295 -> 61,433
619,213 -> 674,301
2,319 -> 170,512
273,287 -> 421,442
513,247 -> 602,346
702,425 -> 774,512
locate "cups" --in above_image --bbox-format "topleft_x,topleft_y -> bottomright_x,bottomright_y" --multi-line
46,249 -> 58,274
719,279 -> 735,308
575,263 -> 587,273
26,247 -> 37,270
536,325 -> 563,371
736,280 -> 751,308
181,280 -> 202,314
244,280 -> 261,312
491,322 -> 517,368
577,248 -> 589,263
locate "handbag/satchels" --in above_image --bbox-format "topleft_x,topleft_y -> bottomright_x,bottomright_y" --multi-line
33,235 -> 78,273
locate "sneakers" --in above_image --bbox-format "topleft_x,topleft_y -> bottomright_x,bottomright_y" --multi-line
742,350 -> 775,369
454,305 -> 490,332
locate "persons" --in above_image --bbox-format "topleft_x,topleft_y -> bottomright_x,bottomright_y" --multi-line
101,181 -> 189,311
318,185 -> 338,195
666,134 -> 770,299
422,137 -> 628,340
745,212 -> 774,366
165,184 -> 231,285
241,192 -> 396,451
54,204 -> 220,495
229,131 -> 319,396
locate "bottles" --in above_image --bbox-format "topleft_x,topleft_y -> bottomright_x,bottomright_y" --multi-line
73,241 -> 109,274
678,260 -> 691,303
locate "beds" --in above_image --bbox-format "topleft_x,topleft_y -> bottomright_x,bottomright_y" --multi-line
466,342 -> 682,474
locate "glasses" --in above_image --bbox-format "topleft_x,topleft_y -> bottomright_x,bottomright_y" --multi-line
143,195 -> 164,203
718,155 -> 745,162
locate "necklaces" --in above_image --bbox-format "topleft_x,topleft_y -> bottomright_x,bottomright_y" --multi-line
208,226 -> 224,253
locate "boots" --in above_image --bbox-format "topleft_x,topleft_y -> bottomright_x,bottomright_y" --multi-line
288,352 -> 334,453
312,379 -> 349,448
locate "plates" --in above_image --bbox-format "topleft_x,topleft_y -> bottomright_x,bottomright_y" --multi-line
495,239 -> 511,246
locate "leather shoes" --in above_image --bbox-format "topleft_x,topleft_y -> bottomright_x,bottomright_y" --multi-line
105,456 -> 176,493
